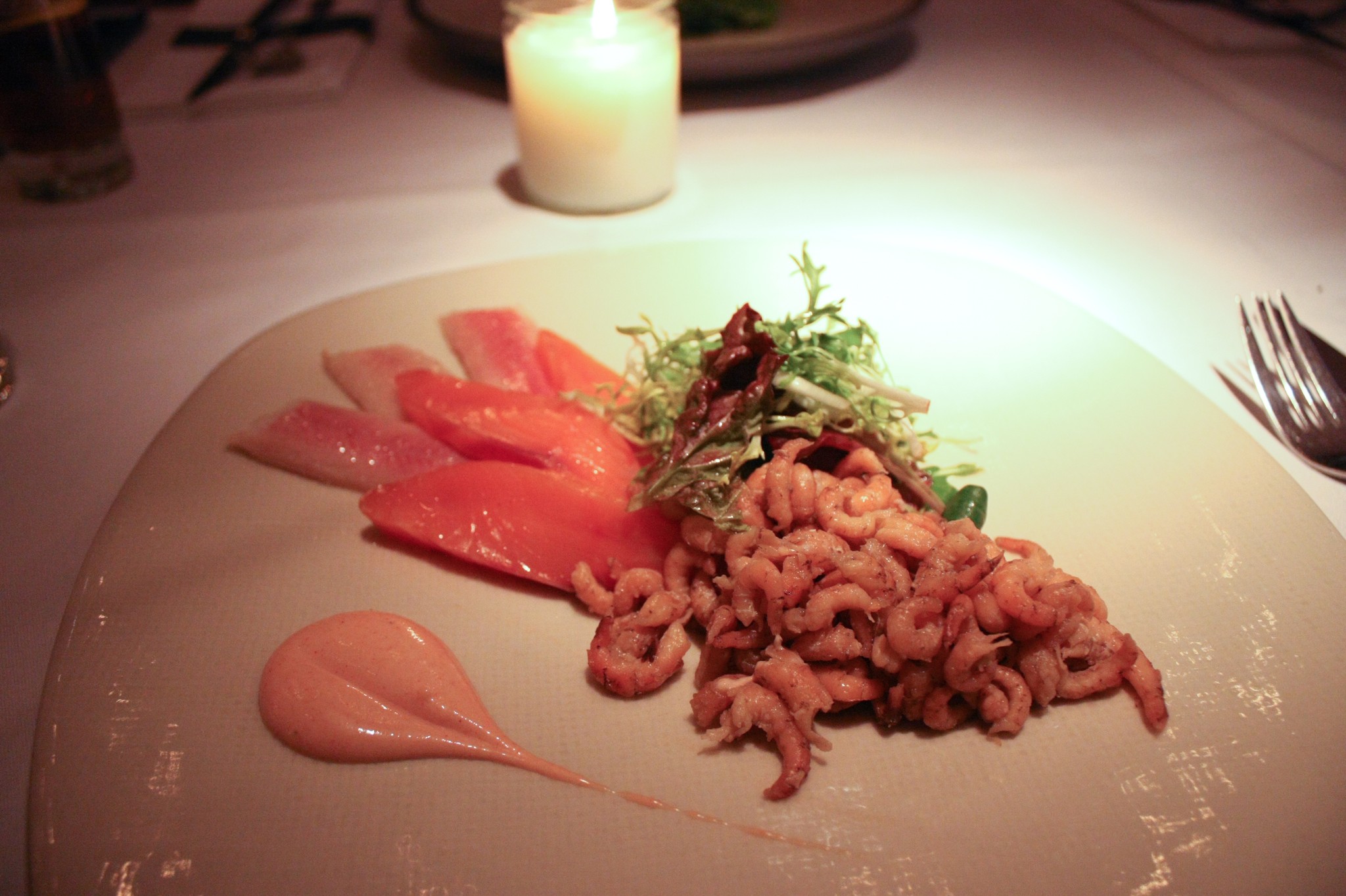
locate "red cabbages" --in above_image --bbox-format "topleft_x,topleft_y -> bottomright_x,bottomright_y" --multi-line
589,244 -> 935,517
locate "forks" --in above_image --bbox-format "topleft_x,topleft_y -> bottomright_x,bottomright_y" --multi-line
1236,282 -> 1345,492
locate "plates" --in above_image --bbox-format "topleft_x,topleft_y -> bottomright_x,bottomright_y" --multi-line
25,252 -> 1346,896
406,2 -> 923,92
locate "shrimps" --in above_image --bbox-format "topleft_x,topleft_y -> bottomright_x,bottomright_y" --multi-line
564,436 -> 1170,806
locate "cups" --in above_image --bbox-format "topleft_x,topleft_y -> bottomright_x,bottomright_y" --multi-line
5,0 -> 135,208
502,1 -> 682,216
